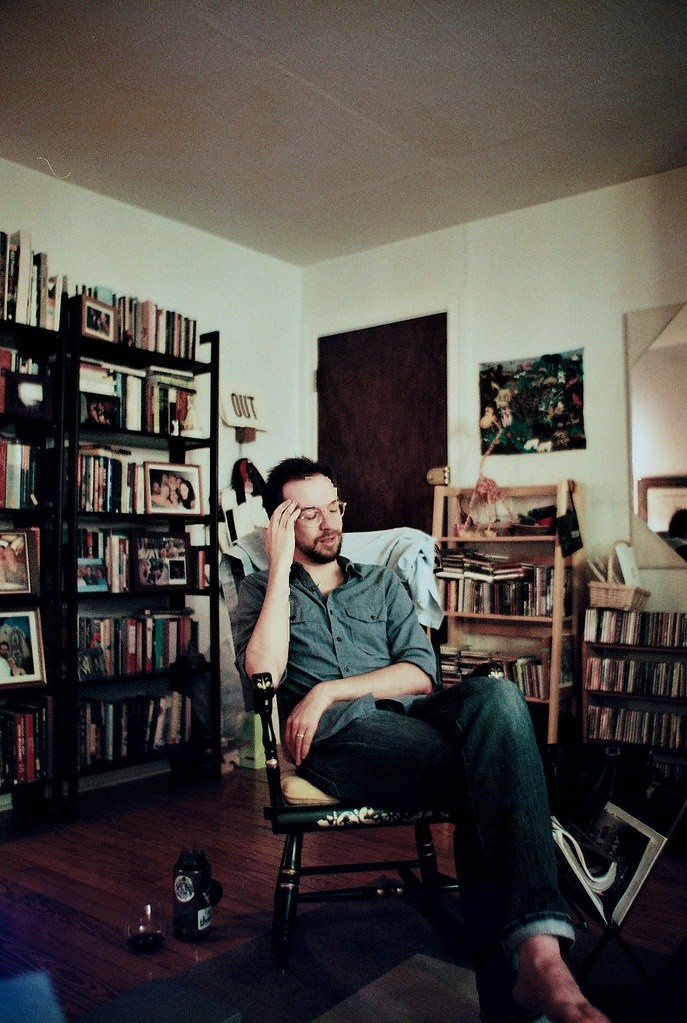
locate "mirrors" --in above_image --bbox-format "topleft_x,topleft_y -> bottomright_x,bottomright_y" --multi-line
625,302 -> 687,567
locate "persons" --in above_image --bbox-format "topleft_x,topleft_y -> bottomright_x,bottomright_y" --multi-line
232,459 -> 611,1023
138,540 -> 186,586
151,470 -> 196,510
77,568 -> 87,585
0,545 -> 33,684
656,507 -> 687,562
98,403 -> 112,425
88,308 -> 98,331
83,565 -> 99,585
98,314 -> 109,336
95,566 -> 107,585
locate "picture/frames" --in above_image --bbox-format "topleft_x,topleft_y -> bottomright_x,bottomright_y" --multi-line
0,531 -> 31,593
143,460 -> 205,515
0,606 -> 48,689
82,295 -> 120,342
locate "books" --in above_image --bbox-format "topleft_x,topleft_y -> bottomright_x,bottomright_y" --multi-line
185,532 -> 206,590
1,442 -> 145,516
78,607 -> 198,767
0,228 -> 201,439
432,544 -> 687,926
77,527 -> 130,593
0,694 -> 54,789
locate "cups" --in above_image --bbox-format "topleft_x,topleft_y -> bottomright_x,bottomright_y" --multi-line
123,898 -> 168,954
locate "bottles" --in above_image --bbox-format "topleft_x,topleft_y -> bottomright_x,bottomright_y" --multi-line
173,848 -> 224,942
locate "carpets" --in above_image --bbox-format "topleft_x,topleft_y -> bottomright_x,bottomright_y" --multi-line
85,870 -> 687,1023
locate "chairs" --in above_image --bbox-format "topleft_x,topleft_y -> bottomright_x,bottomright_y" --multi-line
227,526 -> 460,969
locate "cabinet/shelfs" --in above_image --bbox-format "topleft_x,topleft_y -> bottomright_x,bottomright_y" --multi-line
572,639 -> 687,779
0,293 -> 221,805
430,481 -> 586,791
